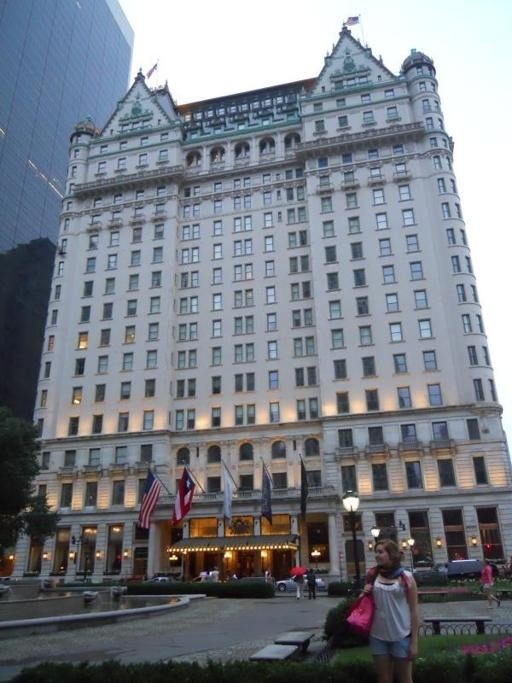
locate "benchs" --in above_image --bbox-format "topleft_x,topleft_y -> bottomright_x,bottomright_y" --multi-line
417,590 -> 449,601
248,644 -> 299,665
423,616 -> 493,635
275,632 -> 315,652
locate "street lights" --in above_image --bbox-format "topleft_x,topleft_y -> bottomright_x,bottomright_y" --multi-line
311,547 -> 322,568
368,523 -> 381,543
70,533 -> 88,571
340,485 -> 363,583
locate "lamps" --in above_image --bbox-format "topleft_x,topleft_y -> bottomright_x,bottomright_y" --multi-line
436,538 -> 443,549
123,550 -> 129,560
69,552 -> 74,560
368,542 -> 373,552
401,539 -> 408,549
96,551 -> 100,560
42,553 -> 48,560
471,536 -> 478,547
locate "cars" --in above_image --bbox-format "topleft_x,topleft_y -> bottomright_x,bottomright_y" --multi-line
274,573 -> 327,593
494,557 -> 512,575
141,576 -> 170,583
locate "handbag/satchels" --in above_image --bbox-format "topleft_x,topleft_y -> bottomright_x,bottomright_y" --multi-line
346,593 -> 374,635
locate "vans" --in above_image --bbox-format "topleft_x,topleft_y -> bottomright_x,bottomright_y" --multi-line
238,574 -> 276,589
412,560 -> 432,572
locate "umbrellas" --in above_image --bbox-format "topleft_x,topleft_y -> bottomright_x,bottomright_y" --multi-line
290,567 -> 306,576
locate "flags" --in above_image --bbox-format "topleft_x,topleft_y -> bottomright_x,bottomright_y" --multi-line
301,462 -> 309,523
171,468 -> 196,528
348,17 -> 358,25
138,469 -> 161,529
148,65 -> 157,77
262,467 -> 272,525
223,468 -> 232,520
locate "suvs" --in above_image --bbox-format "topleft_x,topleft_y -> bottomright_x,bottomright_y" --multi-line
435,558 -> 484,581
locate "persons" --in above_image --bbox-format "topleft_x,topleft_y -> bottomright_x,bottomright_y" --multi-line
440,564 -> 448,580
358,538 -> 420,683
205,571 -> 212,583
267,573 -> 275,592
481,560 -> 500,609
295,575 -> 304,600
212,566 -> 220,582
265,568 -> 269,582
307,569 -> 316,599
